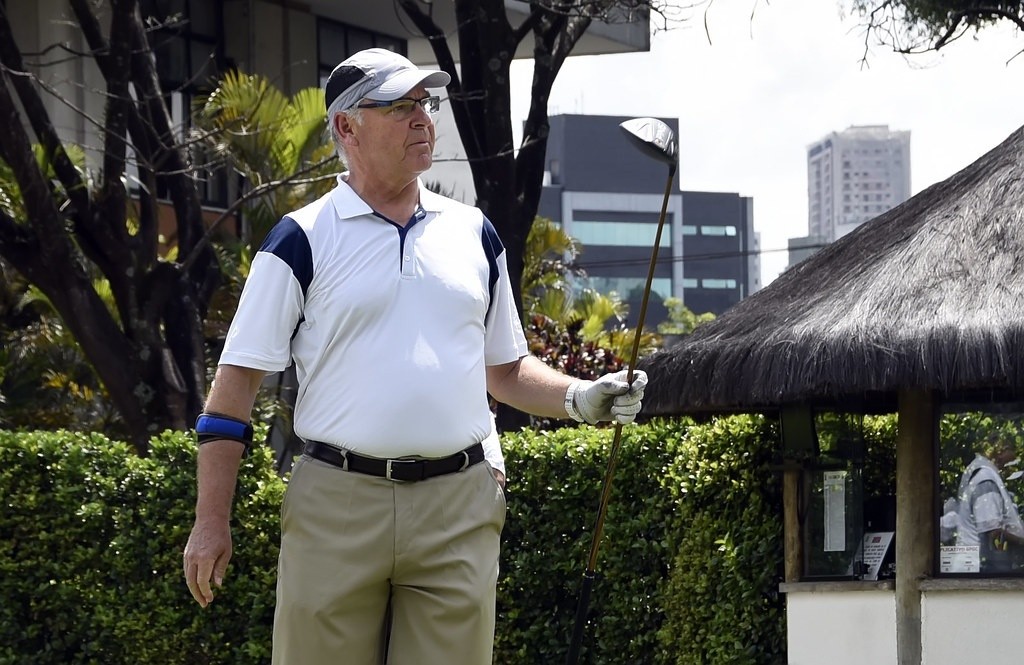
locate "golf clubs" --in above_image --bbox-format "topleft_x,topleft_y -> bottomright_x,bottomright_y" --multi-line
567,117 -> 681,665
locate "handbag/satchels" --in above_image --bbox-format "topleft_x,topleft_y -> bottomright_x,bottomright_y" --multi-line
978,528 -> 1024,578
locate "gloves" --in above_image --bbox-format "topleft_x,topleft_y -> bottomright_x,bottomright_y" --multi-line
574,369 -> 649,425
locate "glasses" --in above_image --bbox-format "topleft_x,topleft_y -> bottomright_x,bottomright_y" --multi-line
357,95 -> 441,121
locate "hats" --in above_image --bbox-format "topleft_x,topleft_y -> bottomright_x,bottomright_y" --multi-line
323,47 -> 451,129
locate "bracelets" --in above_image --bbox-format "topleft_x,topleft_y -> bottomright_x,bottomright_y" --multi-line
564,380 -> 593,423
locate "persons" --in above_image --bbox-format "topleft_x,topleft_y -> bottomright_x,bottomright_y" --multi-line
179,49 -> 647,665
957,432 -> 1024,577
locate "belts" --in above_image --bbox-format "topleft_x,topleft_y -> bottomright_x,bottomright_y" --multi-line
301,439 -> 485,483
